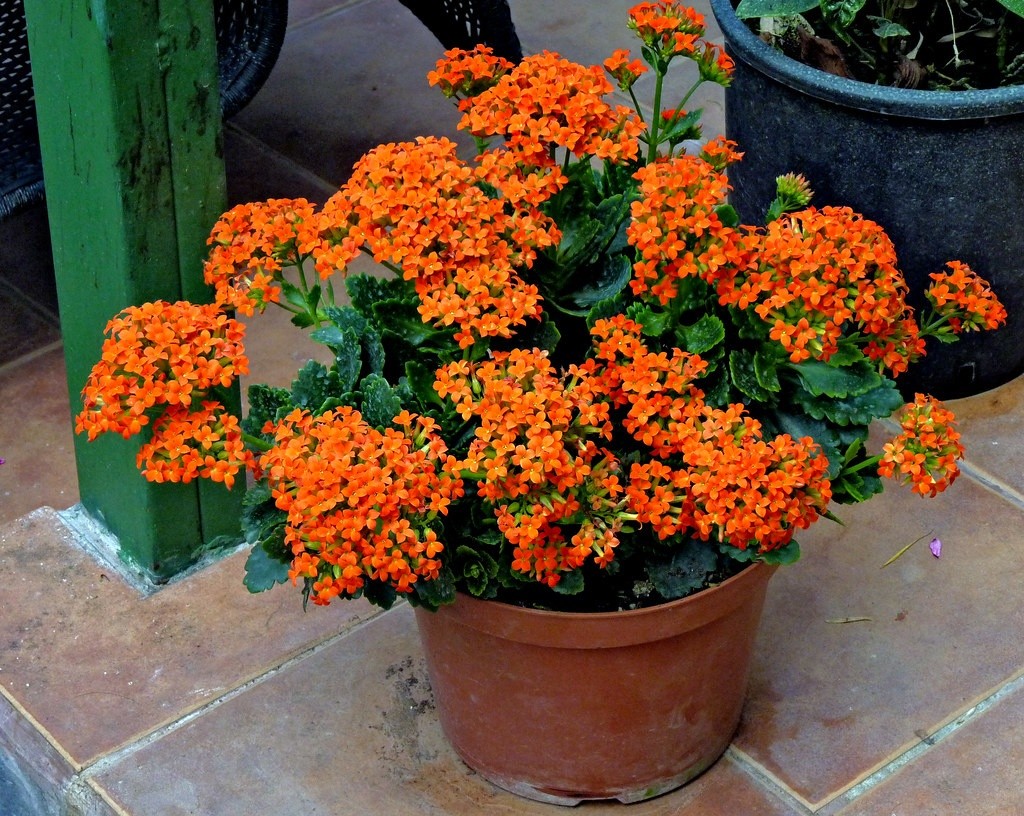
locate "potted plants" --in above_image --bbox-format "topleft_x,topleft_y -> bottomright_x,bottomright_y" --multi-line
709,0 -> 1024,403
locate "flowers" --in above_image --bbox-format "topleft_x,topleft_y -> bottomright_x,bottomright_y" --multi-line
83,0 -> 1000,596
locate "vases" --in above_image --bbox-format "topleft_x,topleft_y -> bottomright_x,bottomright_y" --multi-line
409,565 -> 786,801
